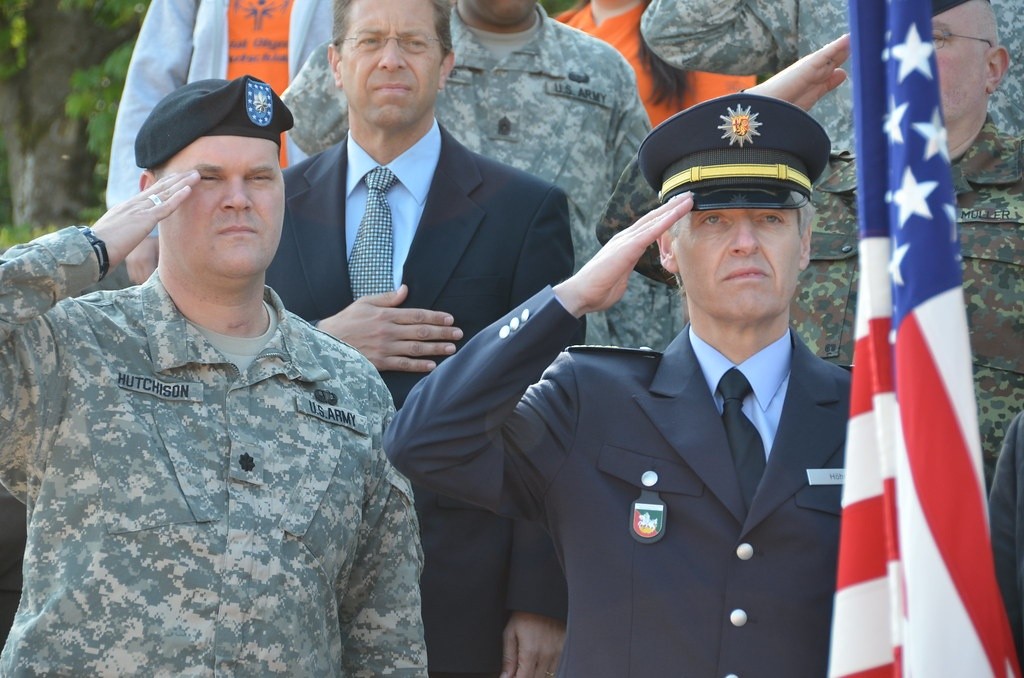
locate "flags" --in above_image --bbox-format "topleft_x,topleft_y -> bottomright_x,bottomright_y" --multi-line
824,0 -> 1021,677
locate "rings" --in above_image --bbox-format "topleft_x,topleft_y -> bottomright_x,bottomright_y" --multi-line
826,59 -> 832,66
546,671 -> 556,677
148,193 -> 161,206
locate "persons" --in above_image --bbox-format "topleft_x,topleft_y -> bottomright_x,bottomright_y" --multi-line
595,0 -> 1023,491
106,0 -> 333,287
260,1 -> 592,678
0,70 -> 438,678
632,0 -> 859,176
275,0 -> 678,348
546,1 -> 760,125
379,93 -> 851,678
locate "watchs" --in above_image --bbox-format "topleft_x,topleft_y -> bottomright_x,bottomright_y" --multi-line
76,225 -> 110,283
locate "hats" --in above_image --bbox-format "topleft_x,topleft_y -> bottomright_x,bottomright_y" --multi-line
135,75 -> 294,168
638,93 -> 832,210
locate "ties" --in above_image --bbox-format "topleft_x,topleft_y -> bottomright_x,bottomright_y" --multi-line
348,166 -> 401,302
717,369 -> 766,514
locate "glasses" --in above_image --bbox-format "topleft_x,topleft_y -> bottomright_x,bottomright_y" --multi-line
336,33 -> 440,50
933,29 -> 992,52
